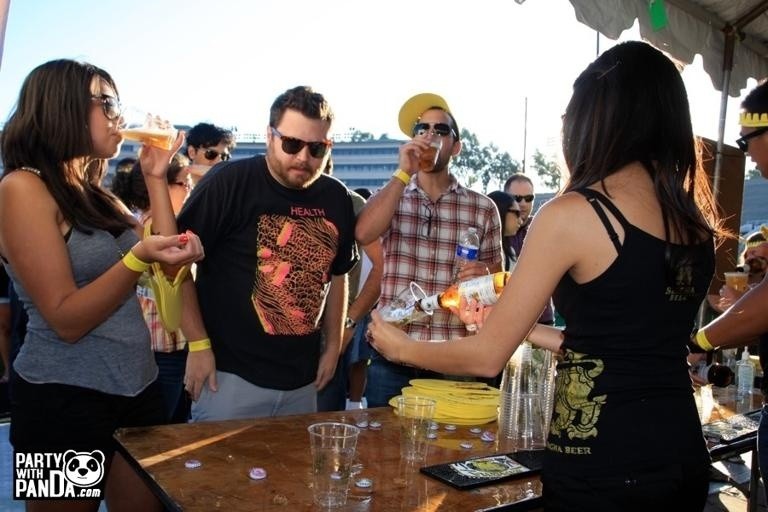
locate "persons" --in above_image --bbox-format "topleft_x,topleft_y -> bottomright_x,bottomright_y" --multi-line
687,79 -> 767,500
1,59 -> 554,512
361,41 -> 728,512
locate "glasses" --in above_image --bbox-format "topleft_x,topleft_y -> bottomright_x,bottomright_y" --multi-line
507,209 -> 521,218
420,202 -> 437,241
271,127 -> 334,159
90,93 -> 123,121
735,126 -> 768,152
172,181 -> 194,192
197,146 -> 230,162
412,122 -> 457,143
512,194 -> 534,203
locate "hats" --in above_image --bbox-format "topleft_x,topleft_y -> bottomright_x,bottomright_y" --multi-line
397,92 -> 460,142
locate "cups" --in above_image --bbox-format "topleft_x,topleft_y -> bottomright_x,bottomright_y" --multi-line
496,341 -> 545,454
392,458 -> 428,508
397,396 -> 437,462
735,346 -> 756,390
377,282 -> 433,327
419,134 -> 443,172
723,271 -> 746,313
307,422 -> 361,508
537,349 -> 555,448
116,105 -> 179,151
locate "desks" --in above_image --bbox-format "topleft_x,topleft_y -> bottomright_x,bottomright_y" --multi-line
113,378 -> 768,512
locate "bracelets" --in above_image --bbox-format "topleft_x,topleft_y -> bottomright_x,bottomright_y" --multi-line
696,328 -> 713,351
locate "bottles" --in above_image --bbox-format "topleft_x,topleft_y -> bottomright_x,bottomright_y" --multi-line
736,391 -> 753,416
415,271 -> 510,312
451,226 -> 480,285
691,361 -> 734,387
